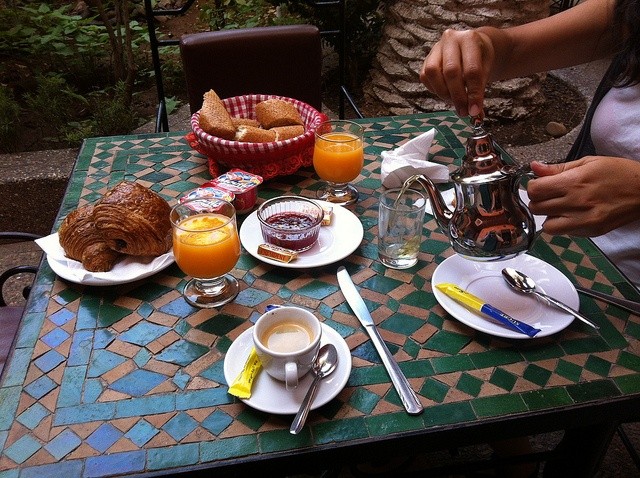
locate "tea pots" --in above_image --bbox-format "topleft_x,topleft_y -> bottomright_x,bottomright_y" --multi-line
416,116 -> 539,263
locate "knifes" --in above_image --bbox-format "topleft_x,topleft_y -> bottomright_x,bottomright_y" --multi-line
337,266 -> 424,414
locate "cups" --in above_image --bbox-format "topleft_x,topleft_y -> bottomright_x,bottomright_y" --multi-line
252,306 -> 323,394
378,187 -> 426,270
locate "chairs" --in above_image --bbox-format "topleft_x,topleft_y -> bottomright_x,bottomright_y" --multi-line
0,231 -> 47,396
145,1 -> 364,133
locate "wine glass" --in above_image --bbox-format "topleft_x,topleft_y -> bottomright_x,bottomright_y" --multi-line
313,121 -> 364,207
170,196 -> 241,310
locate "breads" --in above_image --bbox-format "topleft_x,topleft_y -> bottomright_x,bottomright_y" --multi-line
234,124 -> 276,144
228,117 -> 262,130
57,197 -> 118,273
197,87 -> 235,140
254,99 -> 304,128
93,183 -> 179,259
269,124 -> 306,144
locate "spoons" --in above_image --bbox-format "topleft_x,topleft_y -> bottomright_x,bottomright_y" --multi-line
289,343 -> 339,435
502,268 -> 601,330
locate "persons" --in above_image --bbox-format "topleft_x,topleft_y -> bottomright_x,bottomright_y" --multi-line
416,0 -> 638,241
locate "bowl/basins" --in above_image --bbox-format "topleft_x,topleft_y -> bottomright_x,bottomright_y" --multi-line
190,95 -> 323,170
257,195 -> 324,252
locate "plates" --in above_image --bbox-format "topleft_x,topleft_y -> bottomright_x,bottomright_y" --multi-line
431,252 -> 581,338
239,198 -> 364,269
41,252 -> 176,286
223,322 -> 352,415
